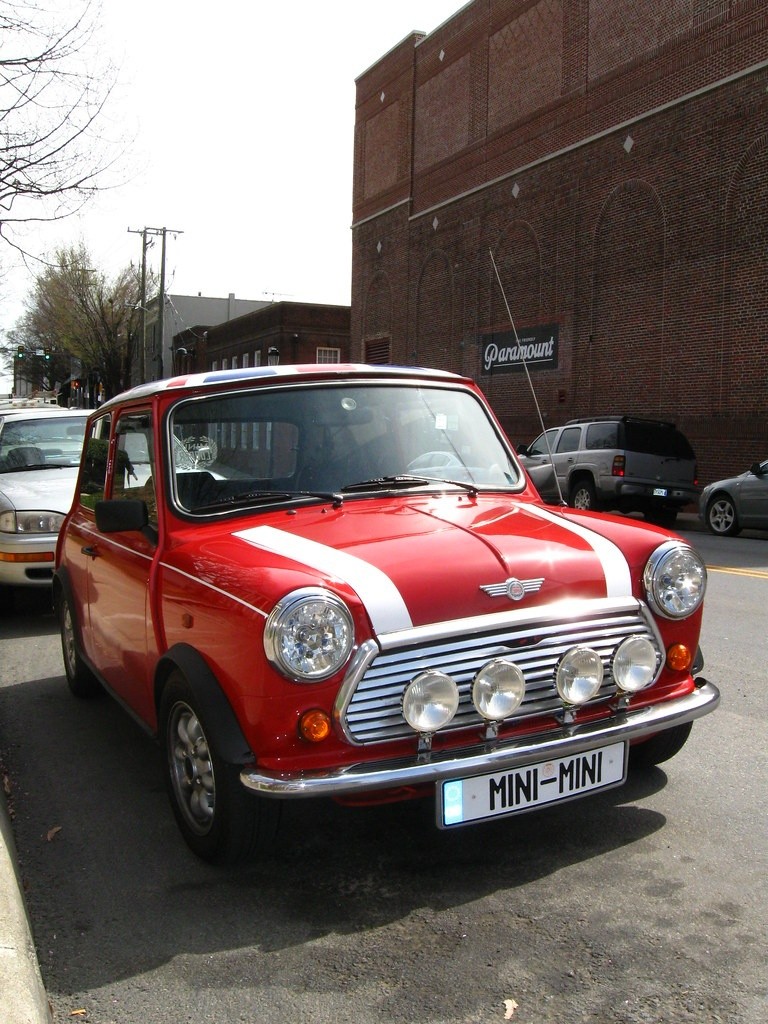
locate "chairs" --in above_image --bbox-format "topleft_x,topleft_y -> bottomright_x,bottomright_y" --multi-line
146,470 -> 213,532
7,446 -> 45,468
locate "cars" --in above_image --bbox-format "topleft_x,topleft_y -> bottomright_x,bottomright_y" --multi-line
699,459 -> 768,537
52,248 -> 721,861
1,409 -> 227,587
407,451 -> 492,484
1,409 -> 96,463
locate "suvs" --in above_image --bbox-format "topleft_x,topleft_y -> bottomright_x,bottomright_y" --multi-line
490,416 -> 697,529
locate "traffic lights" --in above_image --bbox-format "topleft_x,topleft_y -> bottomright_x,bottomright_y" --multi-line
45,348 -> 51,360
18,346 -> 25,360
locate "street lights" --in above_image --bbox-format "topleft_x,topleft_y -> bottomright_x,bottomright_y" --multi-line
123,226 -> 184,381
